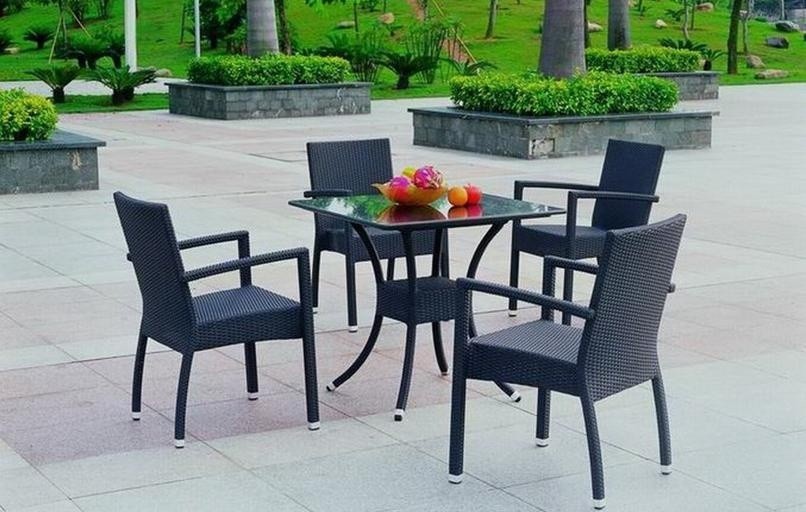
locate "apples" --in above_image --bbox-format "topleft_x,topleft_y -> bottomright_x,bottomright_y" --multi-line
464,205 -> 482,217
464,182 -> 481,205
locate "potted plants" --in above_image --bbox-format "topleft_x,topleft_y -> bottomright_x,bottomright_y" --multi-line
701,48 -> 725,70
371,48 -> 445,90
0,22 -> 159,142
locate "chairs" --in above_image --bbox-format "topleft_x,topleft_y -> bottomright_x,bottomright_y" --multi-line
112,189 -> 322,449
448,211 -> 690,512
306,137 -> 452,333
504,138 -> 668,324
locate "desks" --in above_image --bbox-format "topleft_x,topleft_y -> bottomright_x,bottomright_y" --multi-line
287,192 -> 568,423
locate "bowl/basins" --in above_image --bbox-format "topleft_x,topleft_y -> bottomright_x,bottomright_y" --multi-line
372,181 -> 449,205
373,204 -> 449,227
